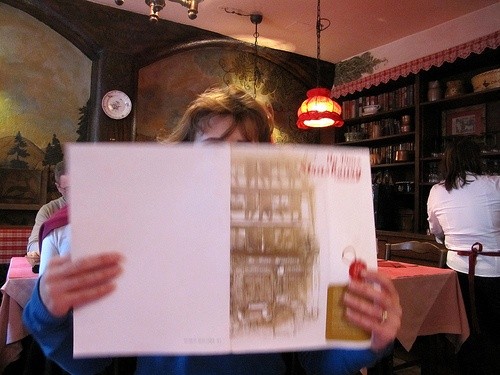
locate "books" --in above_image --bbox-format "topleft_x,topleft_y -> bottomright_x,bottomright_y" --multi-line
343,85 -> 413,120
370,143 -> 414,165
355,118 -> 401,138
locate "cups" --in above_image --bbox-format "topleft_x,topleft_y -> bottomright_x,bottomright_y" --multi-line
363,104 -> 381,114
370,154 -> 381,165
395,150 -> 410,162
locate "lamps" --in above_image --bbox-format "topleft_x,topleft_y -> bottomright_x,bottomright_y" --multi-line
297,0 -> 345,129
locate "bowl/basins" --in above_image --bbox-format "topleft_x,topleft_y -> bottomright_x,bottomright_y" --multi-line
344,132 -> 363,142
25,256 -> 39,266
394,182 -> 414,192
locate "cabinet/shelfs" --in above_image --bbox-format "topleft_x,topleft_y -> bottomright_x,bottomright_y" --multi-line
329,45 -> 500,238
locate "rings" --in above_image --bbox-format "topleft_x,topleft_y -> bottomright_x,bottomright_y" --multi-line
381,311 -> 387,322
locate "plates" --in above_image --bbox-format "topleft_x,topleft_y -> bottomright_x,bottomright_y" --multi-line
102,90 -> 132,120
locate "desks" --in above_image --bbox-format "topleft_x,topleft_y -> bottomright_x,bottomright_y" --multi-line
378,258 -> 469,352
0,255 -> 46,367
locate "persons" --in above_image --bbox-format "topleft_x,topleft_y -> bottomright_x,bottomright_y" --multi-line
26,161 -> 70,266
23,85 -> 402,375
39,206 -> 70,277
426,136 -> 500,374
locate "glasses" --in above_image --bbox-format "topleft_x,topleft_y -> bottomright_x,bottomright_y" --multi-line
59,186 -> 68,193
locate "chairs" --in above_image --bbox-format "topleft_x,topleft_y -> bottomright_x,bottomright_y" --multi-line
384,242 -> 447,270
